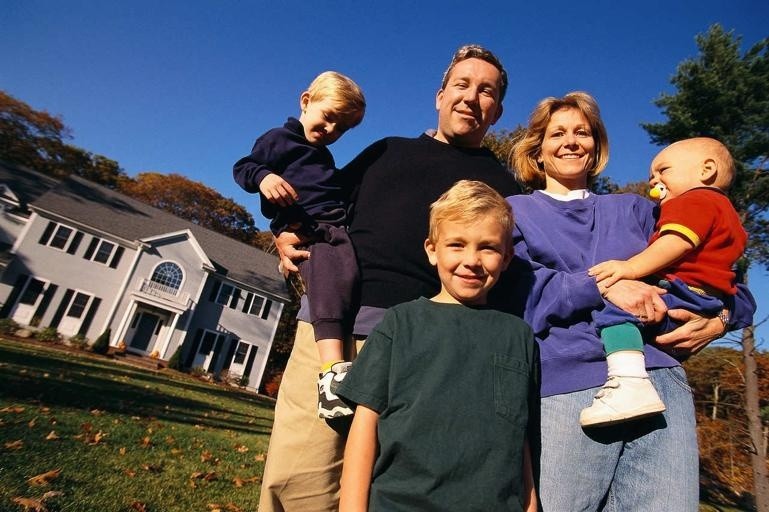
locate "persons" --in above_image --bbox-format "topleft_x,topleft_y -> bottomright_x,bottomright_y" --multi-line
504,91 -> 758,511
581,136 -> 749,428
335,180 -> 540,511
259,45 -> 529,512
232,70 -> 356,419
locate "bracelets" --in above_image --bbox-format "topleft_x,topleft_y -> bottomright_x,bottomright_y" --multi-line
715,310 -> 730,329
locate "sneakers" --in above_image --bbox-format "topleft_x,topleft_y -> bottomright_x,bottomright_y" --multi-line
318,362 -> 354,418
580,378 -> 666,427
